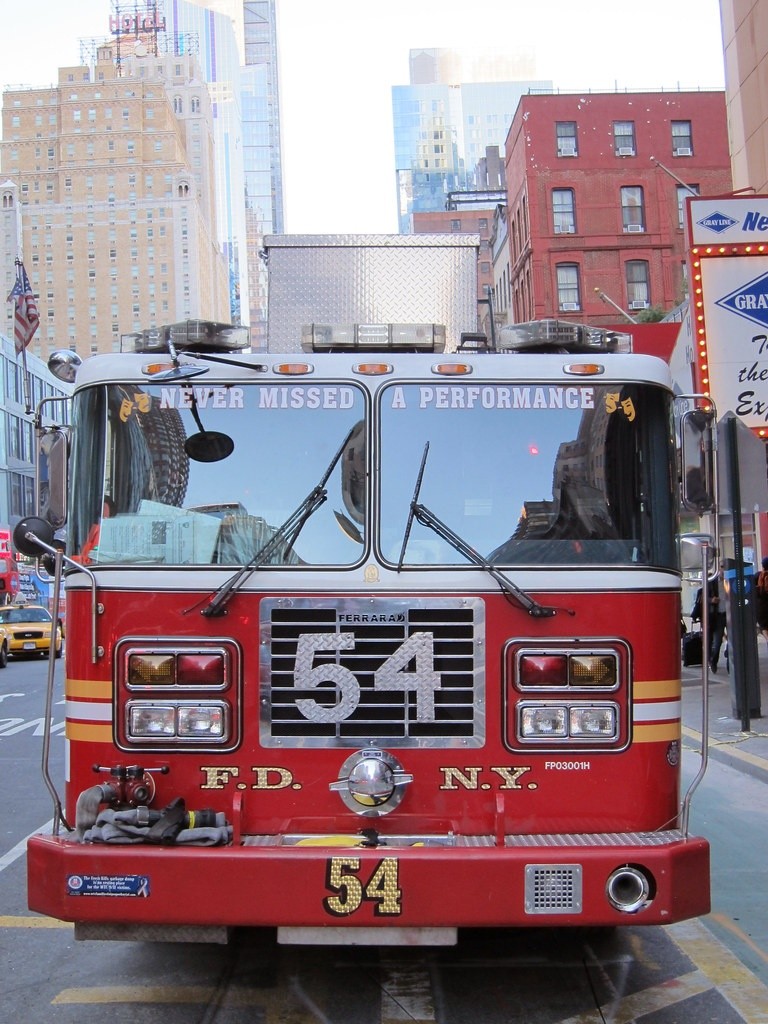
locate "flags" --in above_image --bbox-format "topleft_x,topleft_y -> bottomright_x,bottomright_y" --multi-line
6,261 -> 41,364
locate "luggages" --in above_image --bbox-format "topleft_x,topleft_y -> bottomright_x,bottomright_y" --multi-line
683,621 -> 703,667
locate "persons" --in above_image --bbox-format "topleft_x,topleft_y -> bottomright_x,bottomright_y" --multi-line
690,565 -> 730,674
757,557 -> 768,645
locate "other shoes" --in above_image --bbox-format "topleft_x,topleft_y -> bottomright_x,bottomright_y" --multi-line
710,658 -> 718,674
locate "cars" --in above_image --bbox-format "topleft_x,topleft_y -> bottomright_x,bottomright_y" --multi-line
0,592 -> 63,659
0,627 -> 10,669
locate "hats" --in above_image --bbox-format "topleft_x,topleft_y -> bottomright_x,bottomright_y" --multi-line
762,556 -> 768,566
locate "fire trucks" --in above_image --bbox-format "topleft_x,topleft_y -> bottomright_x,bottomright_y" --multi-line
13,230 -> 721,946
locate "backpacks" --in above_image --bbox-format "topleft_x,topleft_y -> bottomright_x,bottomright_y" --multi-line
757,571 -> 768,600
